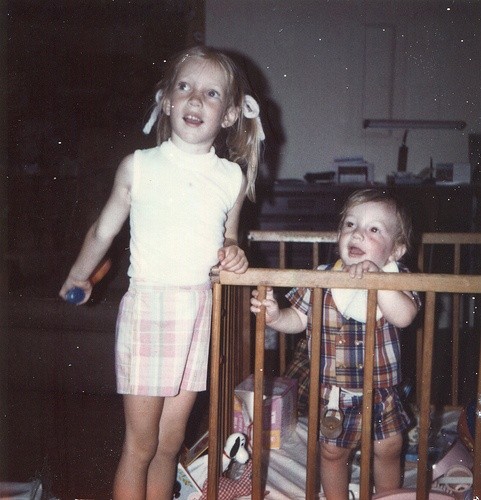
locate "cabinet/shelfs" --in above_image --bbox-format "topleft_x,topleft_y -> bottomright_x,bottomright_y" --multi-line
256,178 -> 481,275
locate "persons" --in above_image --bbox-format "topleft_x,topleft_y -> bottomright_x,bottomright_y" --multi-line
55,45 -> 267,500
250,188 -> 423,499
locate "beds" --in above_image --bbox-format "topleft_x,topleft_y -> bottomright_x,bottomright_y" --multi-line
205,231 -> 481,500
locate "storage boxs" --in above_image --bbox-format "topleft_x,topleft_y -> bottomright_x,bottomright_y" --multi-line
232,373 -> 297,451
